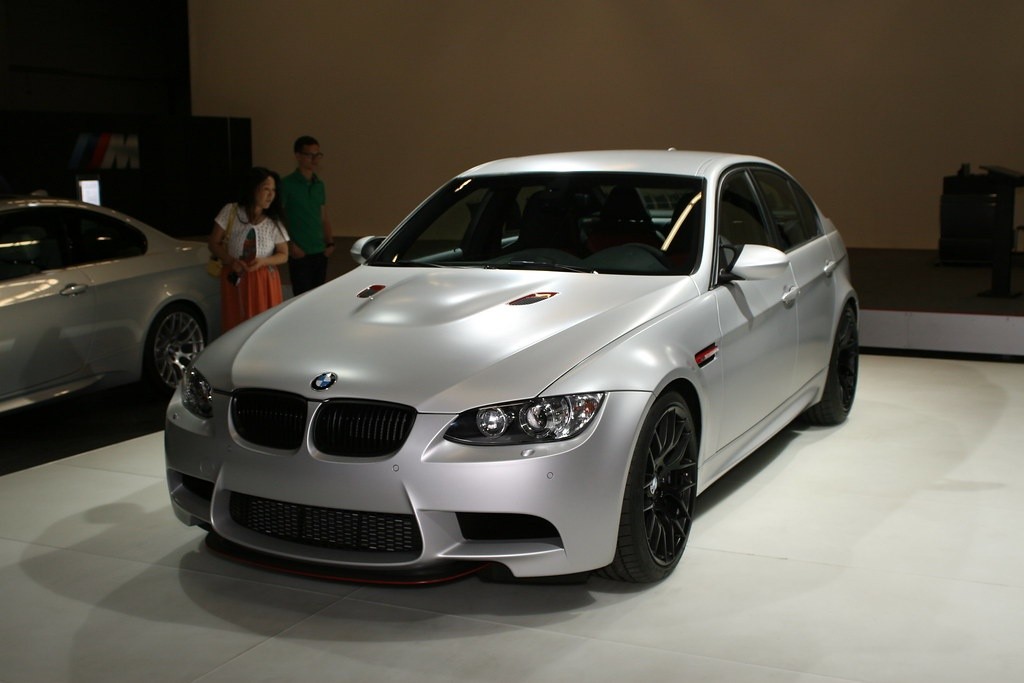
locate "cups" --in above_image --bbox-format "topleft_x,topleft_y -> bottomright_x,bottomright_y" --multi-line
961,163 -> 969,175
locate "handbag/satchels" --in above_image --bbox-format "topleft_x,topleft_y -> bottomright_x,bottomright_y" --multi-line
207,202 -> 238,277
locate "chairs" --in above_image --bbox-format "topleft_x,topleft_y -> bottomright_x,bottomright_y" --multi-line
582,185 -> 662,256
671,189 -> 772,266
500,190 -> 582,256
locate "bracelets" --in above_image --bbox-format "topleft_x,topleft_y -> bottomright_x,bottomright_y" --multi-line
325,243 -> 333,246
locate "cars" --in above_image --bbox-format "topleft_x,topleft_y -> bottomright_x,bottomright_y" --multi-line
165,147 -> 864,587
0,195 -> 221,423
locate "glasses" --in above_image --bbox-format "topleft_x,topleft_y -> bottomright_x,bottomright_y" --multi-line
302,152 -> 323,159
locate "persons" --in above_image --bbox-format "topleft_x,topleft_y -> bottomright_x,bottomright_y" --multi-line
273,136 -> 335,298
208,167 -> 289,338
